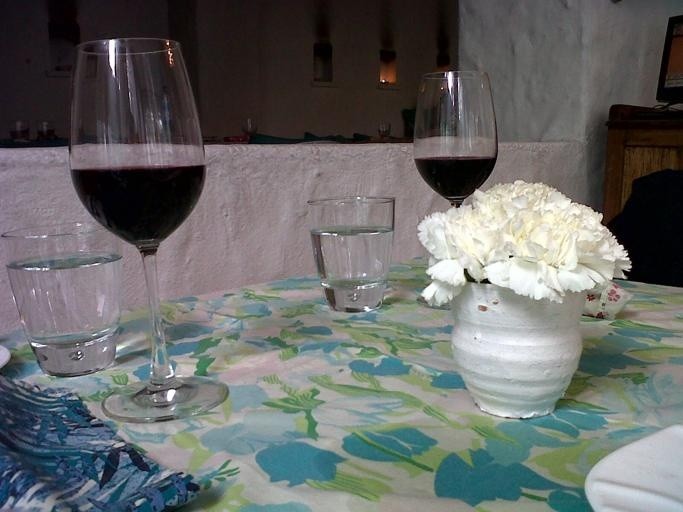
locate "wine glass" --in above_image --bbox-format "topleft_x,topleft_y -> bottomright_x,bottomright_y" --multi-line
410,69 -> 501,312
64,34 -> 233,425
239,117 -> 258,143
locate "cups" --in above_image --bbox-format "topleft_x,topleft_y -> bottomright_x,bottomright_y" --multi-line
35,118 -> 56,141
6,118 -> 31,142
0,219 -> 125,380
376,119 -> 390,139
305,193 -> 397,317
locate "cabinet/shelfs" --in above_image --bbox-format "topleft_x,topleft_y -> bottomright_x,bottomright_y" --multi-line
603,121 -> 683,236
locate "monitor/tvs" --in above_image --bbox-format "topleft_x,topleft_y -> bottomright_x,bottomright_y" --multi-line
655,13 -> 683,109
